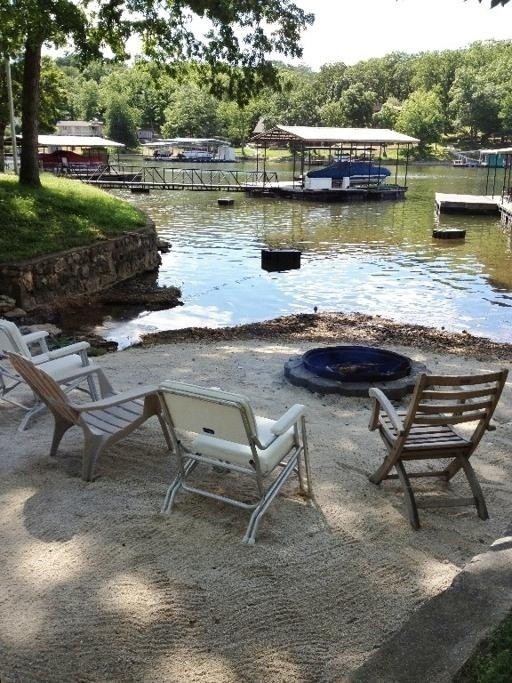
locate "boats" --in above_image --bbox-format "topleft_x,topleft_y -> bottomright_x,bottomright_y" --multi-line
453,148 -> 512,167
140,138 -> 238,162
14,134 -> 142,181
241,124 -> 421,200
